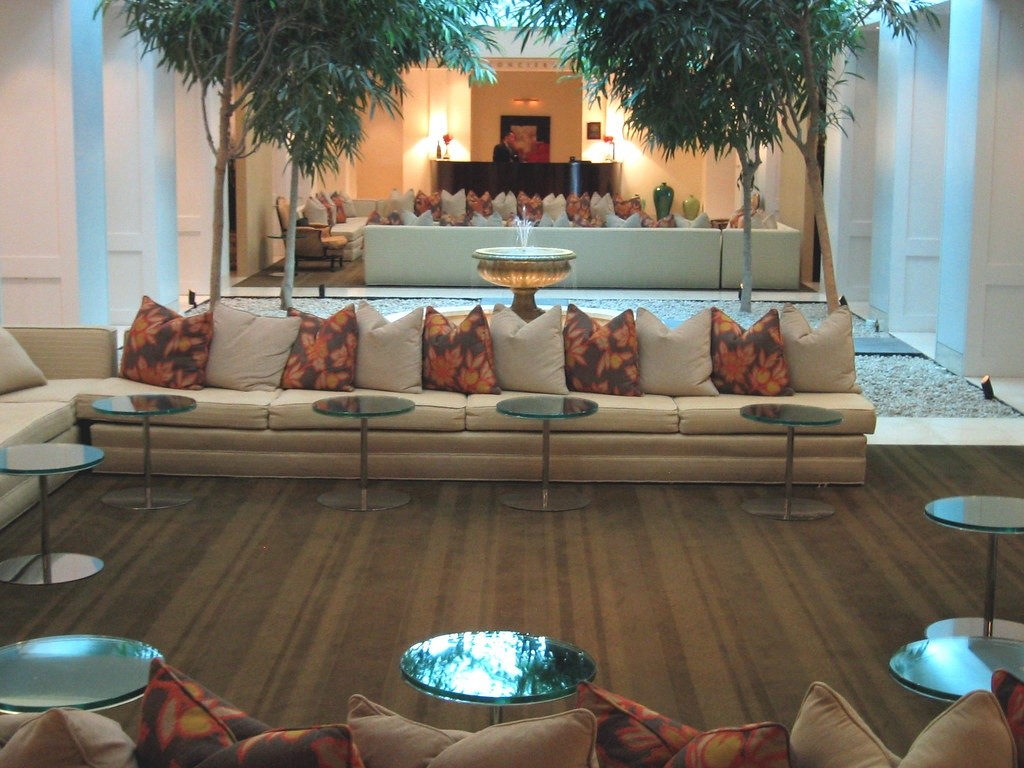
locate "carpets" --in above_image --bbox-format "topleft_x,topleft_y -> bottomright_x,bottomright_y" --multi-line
0,438 -> 1024,758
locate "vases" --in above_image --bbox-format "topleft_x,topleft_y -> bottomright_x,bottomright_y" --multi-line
653,182 -> 674,219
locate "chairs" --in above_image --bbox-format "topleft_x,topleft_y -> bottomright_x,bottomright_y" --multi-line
276,195 -> 347,276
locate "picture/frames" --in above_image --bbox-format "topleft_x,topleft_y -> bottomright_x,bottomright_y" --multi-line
586,120 -> 603,141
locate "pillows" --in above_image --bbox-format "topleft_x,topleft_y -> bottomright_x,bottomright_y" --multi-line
490,302 -> 572,395
278,303 -> 359,393
634,307 -> 721,398
782,302 -> 863,395
138,657 -> 363,768
0,326 -> 49,395
120,295 -> 216,392
576,677 -> 790,768
351,298 -> 426,397
203,298 -> 303,393
989,668 -> 1023,768
710,304 -> 794,397
787,679 -> 1018,768
344,691 -> 600,768
0,706 -> 139,768
419,303 -> 504,397
306,184 -> 782,230
563,303 -> 646,398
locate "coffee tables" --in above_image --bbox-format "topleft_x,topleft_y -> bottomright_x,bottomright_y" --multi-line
397,626 -> 596,725
494,396 -> 600,514
920,493 -> 1024,650
0,632 -> 168,722
740,402 -> 846,524
1,445 -> 107,589
89,391 -> 200,509
886,633 -> 1024,711
308,396 -> 420,511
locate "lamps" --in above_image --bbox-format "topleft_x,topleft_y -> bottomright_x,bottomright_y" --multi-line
441,133 -> 455,160
603,134 -> 619,163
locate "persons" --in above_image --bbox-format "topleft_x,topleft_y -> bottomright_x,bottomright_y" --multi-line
492,130 -> 527,199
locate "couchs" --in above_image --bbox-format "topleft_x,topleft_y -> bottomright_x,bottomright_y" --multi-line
363,220 -> 721,292
719,222 -> 805,292
304,197 -> 377,266
81,308 -> 880,488
0,324 -> 119,523
